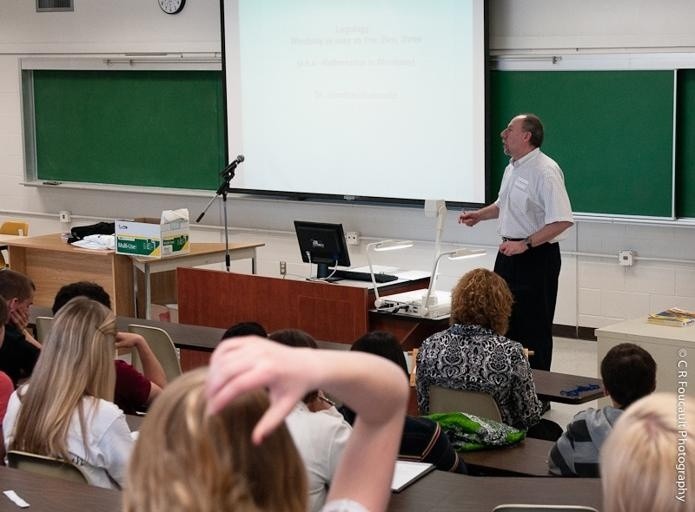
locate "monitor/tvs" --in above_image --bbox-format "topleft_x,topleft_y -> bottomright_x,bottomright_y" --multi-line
294,221 -> 350,282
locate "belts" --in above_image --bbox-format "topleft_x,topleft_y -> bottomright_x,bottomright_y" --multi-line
500,236 -> 527,244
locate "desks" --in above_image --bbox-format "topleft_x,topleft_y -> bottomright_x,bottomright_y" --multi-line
594,309 -> 695,412
130,243 -> 264,320
1,466 -> 126,511
24,305 -> 608,418
176,265 -> 450,351
0,232 -> 191,356
121,414 -> 601,512
125,413 -> 555,476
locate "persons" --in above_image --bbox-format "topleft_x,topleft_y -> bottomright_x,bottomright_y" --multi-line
0,270 -> 42,390
221,321 -> 268,344
546,342 -> 657,478
415,268 -> 562,442
125,334 -> 410,512
598,392 -> 695,512
2,295 -> 141,493
458,114 -> 575,417
52,282 -> 167,415
267,327 -> 354,512
0,298 -> 14,468
338,331 -> 477,477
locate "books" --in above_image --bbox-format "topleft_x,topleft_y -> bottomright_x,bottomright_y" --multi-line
648,307 -> 694,327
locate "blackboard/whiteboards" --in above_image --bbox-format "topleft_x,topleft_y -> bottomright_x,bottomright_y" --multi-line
676,68 -> 695,218
31,70 -> 675,218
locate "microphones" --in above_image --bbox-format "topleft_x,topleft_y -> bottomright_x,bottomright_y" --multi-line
220,154 -> 244,177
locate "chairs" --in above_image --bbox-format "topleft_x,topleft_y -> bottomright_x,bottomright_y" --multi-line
35,316 -> 54,344
0,220 -> 28,271
126,324 -> 183,388
6,449 -> 88,481
428,383 -> 502,423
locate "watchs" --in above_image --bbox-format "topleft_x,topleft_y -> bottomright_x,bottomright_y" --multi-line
526,236 -> 534,250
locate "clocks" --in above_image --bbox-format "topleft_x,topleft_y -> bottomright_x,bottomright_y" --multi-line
158,0 -> 185,15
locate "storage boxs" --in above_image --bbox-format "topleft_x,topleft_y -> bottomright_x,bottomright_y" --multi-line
116,208 -> 190,259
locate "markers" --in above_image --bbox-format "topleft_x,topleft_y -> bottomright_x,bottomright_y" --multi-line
43,181 -> 62,185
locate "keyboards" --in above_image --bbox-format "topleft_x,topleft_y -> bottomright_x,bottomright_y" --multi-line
328,269 -> 398,283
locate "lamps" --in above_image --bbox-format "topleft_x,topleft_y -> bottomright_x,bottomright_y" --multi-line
365,240 -> 414,300
422,248 -> 489,315
